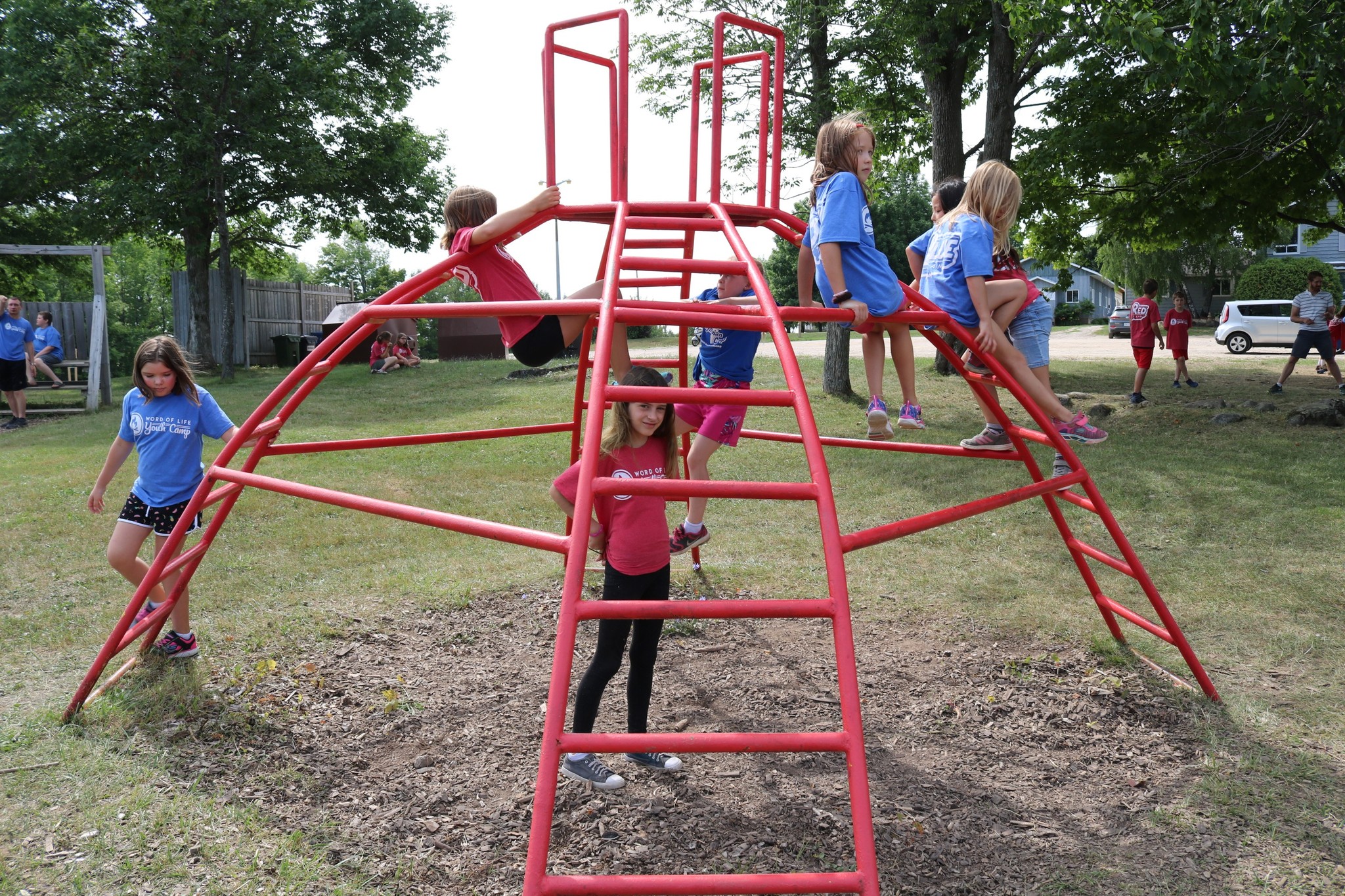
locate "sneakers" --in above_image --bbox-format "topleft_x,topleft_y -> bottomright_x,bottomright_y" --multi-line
1172,380 -> 1182,388
155,630 -> 198,659
370,369 -> 387,373
0,416 -> 18,428
897,401 -> 925,429
1184,377 -> 1199,387
1316,364 -> 1328,371
865,395 -> 894,441
1129,393 -> 1149,404
1051,411 -> 1108,444
6,418 -> 28,429
128,603 -> 158,629
960,428 -> 1013,451
1339,385 -> 1345,396
963,350 -> 993,375
1052,453 -> 1074,489
561,753 -> 625,790
626,752 -> 683,771
612,366 -> 673,386
1266,383 -> 1282,393
669,524 -> 710,556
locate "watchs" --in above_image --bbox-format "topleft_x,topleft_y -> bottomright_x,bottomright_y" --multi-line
30,363 -> 36,366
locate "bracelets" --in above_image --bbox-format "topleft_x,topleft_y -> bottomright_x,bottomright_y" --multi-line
833,289 -> 847,298
832,292 -> 852,304
589,524 -> 604,537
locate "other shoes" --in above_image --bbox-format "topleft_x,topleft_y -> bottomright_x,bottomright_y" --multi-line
414,365 -> 421,368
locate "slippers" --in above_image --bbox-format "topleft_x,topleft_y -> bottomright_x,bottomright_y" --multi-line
27,381 -> 37,387
51,381 -> 65,389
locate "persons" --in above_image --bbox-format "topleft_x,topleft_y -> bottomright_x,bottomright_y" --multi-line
1129,278 -> 1165,405
794,112 -> 1110,495
86,336 -> 280,660
1268,271 -> 1345,394
0,294 -> 65,429
1164,291 -> 1199,389
370,332 -> 421,374
442,185 -> 674,388
666,256 -> 771,556
550,366 -> 682,789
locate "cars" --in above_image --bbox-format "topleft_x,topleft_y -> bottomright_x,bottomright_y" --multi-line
1107,305 -> 1132,338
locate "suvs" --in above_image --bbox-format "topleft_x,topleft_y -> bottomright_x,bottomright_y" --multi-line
1214,299 -> 1300,354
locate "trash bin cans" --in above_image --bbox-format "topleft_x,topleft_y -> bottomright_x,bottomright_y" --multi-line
300,335 -> 318,361
311,332 -> 322,346
270,334 -> 301,367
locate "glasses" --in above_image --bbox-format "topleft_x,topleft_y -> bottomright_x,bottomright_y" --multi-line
400,338 -> 406,340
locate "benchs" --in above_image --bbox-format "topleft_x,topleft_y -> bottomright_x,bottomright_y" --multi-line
0,359 -> 89,415
